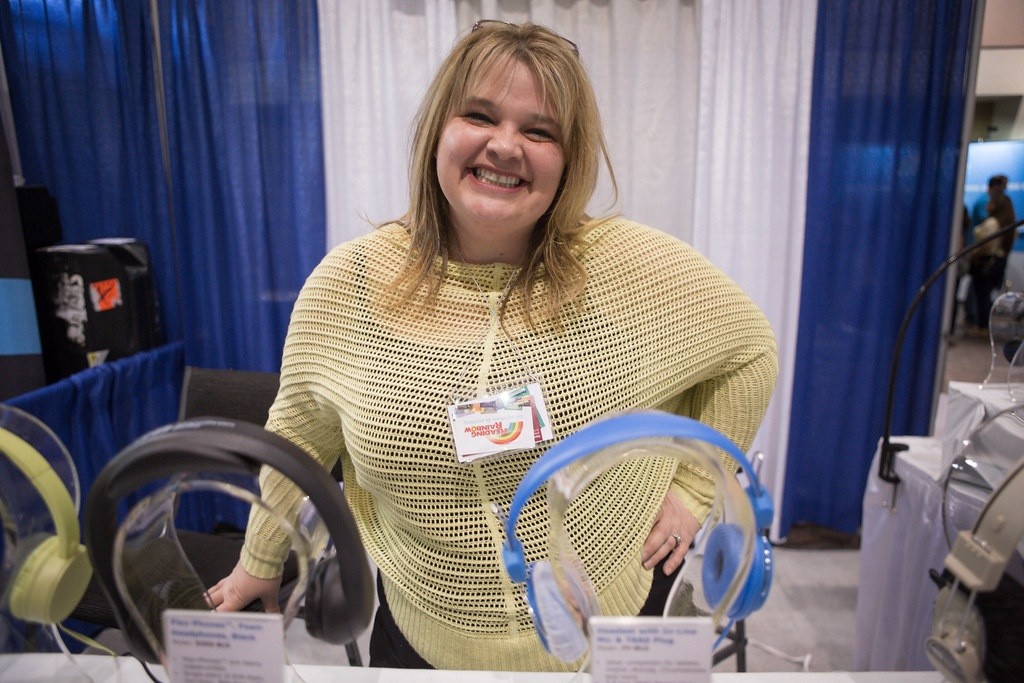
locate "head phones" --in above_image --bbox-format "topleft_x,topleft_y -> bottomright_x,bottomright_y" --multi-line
503,411 -> 774,665
86,415 -> 376,666
0,427 -> 94,625
924,456 -> 1024,683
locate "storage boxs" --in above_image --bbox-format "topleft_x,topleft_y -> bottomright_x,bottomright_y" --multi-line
33,236 -> 166,380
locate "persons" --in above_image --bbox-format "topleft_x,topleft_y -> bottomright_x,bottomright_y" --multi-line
947,175 -> 1016,335
204,17 -> 778,672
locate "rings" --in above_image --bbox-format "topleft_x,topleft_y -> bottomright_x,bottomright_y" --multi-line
671,531 -> 682,547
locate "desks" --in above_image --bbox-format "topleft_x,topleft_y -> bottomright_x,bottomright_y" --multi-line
855,435 -> 992,672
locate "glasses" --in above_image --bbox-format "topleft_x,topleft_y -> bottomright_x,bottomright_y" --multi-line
472,19 -> 579,60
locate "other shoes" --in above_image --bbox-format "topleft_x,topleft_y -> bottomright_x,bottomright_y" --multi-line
955,322 -> 989,337
